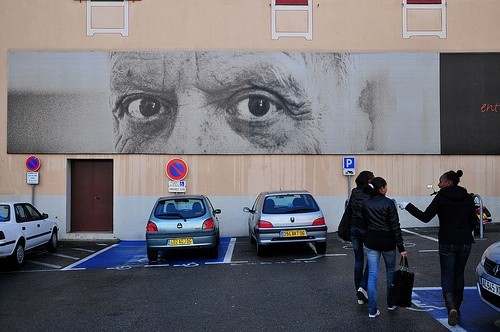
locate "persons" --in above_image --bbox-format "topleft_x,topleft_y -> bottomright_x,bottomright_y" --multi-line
349,171 -> 375,305
398,170 -> 474,324
108,51 -> 397,154
362,176 -> 407,318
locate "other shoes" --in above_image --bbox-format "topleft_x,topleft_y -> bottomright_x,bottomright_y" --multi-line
358,298 -> 364,304
448,308 -> 458,326
369,310 -> 380,318
357,287 -> 368,303
388,306 -> 398,310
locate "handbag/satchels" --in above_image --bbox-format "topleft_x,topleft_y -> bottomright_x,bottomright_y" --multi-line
338,197 -> 353,242
388,256 -> 414,307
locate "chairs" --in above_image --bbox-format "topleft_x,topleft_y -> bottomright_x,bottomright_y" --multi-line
292,198 -> 305,207
166,203 -> 176,212
265,198 -> 276,208
192,202 -> 202,212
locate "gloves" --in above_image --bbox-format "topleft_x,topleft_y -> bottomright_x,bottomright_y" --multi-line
396,202 -> 408,210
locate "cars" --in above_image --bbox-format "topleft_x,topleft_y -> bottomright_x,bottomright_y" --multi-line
476,240 -> 499,312
145,193 -> 222,261
0,201 -> 60,264
243,189 -> 329,256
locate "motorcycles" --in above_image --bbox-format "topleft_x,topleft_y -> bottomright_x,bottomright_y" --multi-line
426,184 -> 492,234
